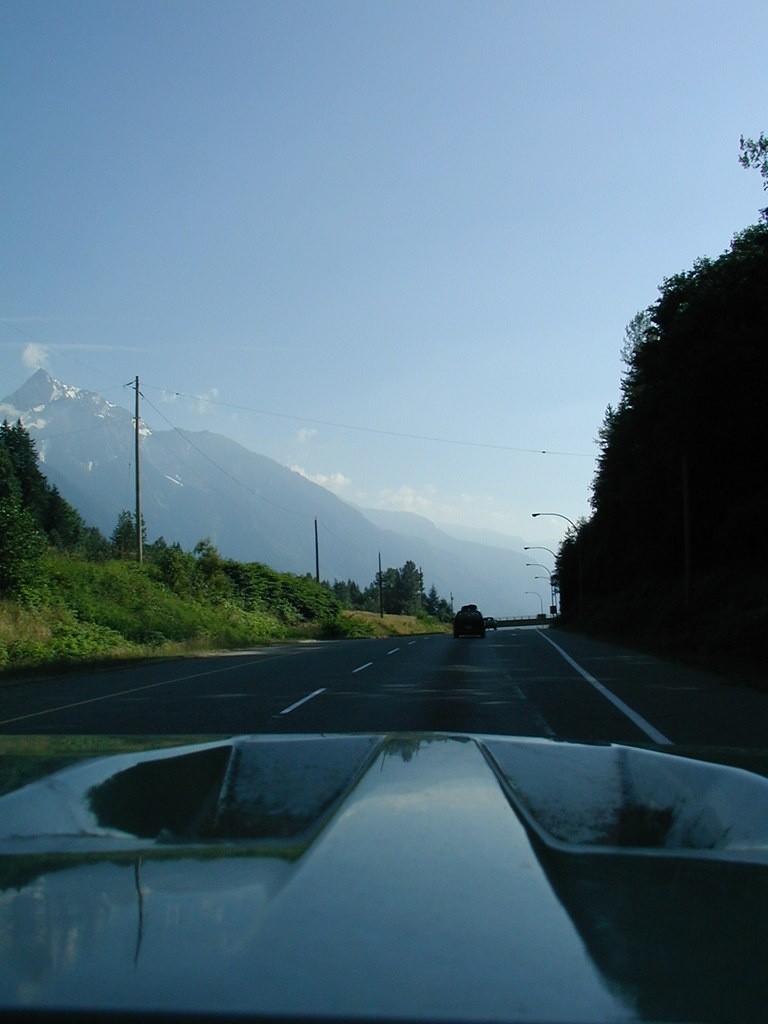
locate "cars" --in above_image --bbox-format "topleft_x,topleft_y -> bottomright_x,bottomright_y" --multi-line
483,617 -> 498,631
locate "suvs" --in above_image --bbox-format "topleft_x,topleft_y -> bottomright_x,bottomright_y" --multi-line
454,605 -> 487,638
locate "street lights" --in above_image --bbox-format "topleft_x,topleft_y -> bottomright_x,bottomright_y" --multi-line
525,562 -> 559,619
